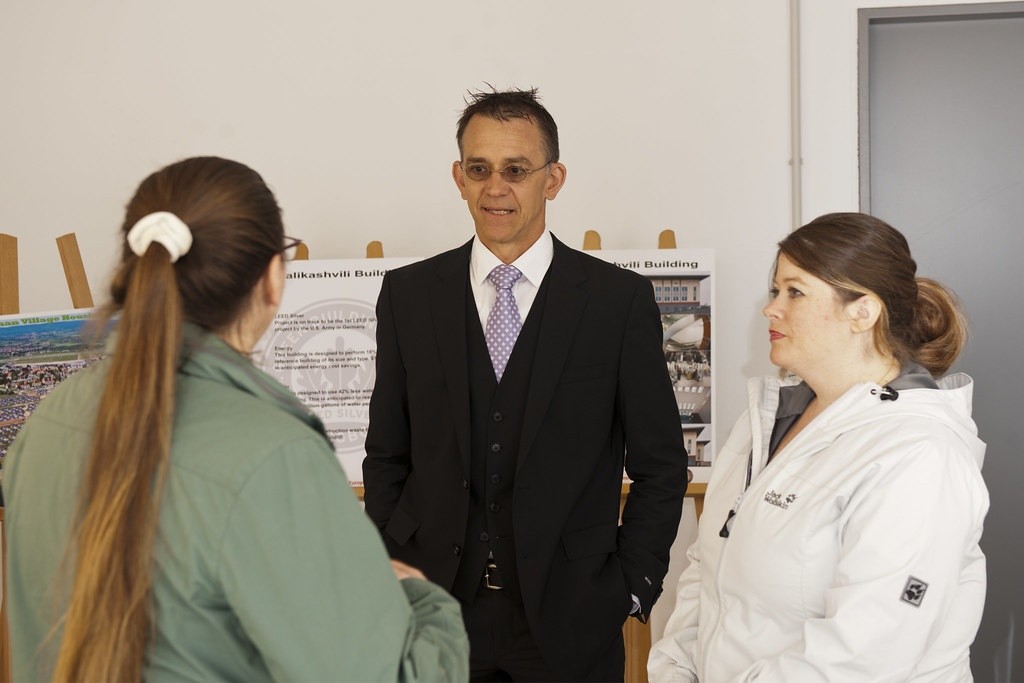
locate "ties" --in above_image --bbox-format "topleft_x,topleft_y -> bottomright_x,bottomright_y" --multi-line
484,264 -> 523,384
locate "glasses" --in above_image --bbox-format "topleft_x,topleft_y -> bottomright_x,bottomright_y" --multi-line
459,159 -> 551,181
279,236 -> 300,257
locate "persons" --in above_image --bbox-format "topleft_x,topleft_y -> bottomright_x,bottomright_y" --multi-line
645,213 -> 990,683
0,155 -> 472,683
361,83 -> 686,683
